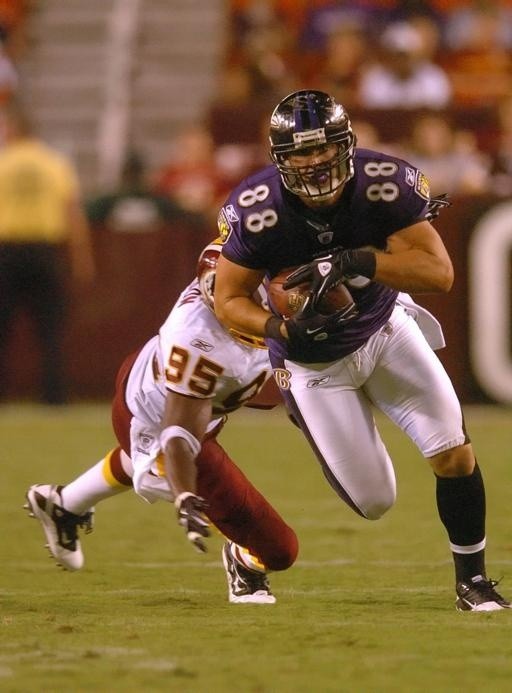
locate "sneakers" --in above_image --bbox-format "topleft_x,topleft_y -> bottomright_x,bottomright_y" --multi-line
453,574 -> 512,612
21,481 -> 96,572
221,542 -> 278,604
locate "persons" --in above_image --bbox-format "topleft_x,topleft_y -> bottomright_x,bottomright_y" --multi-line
24,231 -> 299,605
0,97 -> 98,406
210,90 -> 510,618
81,0 -> 511,227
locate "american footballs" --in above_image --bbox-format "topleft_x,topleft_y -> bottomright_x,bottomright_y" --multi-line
267,266 -> 355,325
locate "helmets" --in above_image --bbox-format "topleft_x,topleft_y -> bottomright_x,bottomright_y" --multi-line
196,234 -> 272,319
267,89 -> 357,201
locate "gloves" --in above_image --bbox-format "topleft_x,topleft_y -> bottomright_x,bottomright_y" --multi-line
173,491 -> 214,556
278,248 -> 361,345
